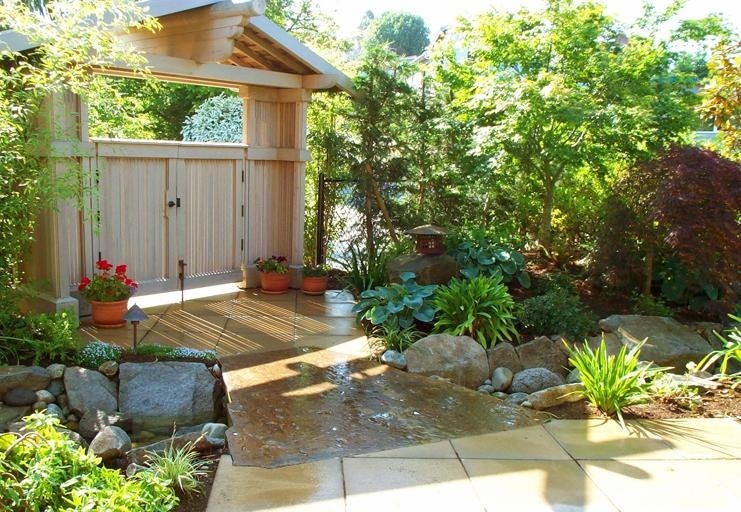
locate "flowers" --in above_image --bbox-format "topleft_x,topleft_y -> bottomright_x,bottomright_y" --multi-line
252,255 -> 288,274
76,259 -> 139,301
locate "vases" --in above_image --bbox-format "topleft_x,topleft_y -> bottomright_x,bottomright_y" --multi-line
259,272 -> 288,295
90,301 -> 127,328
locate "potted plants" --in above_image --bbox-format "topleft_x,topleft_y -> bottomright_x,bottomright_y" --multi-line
302,264 -> 331,296
